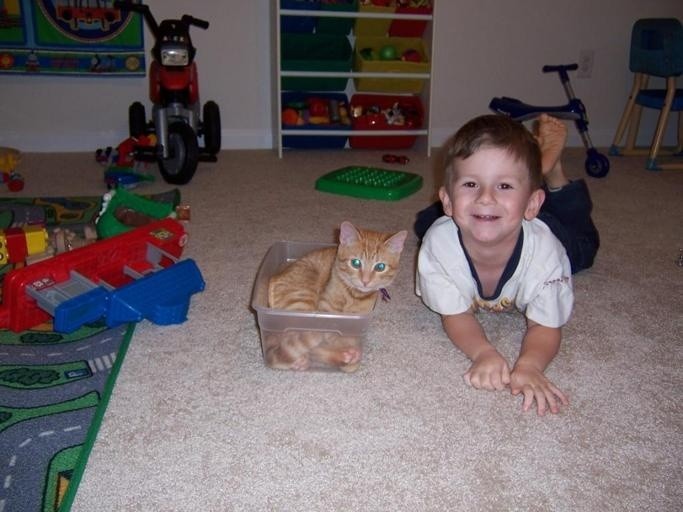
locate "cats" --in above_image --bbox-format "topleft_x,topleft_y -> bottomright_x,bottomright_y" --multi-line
266,220 -> 408,374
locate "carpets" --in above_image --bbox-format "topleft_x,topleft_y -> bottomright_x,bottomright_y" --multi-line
0,183 -> 185,511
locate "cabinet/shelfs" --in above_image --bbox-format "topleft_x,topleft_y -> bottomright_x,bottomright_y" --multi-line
268,1 -> 437,160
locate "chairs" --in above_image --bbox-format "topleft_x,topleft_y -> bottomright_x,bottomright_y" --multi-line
609,17 -> 683,172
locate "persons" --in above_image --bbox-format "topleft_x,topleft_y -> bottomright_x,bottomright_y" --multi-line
412,110 -> 605,417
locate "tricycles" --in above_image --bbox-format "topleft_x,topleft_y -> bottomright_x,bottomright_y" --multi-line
106,2 -> 221,187
